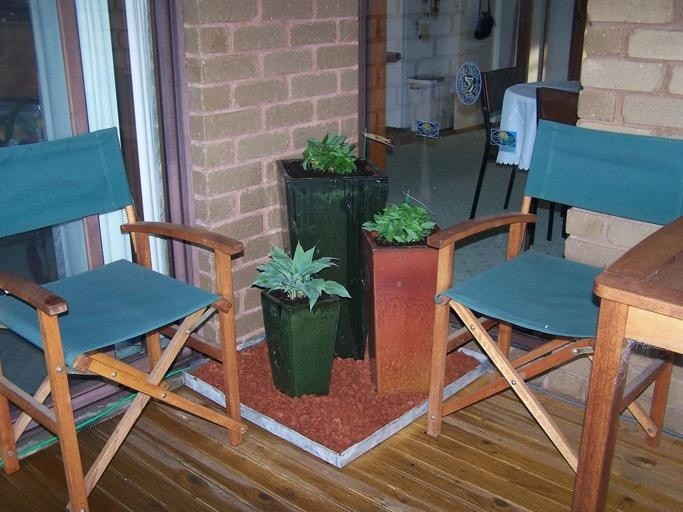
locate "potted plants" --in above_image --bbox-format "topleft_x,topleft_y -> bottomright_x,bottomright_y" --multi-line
275,133 -> 390,360
359,198 -> 443,397
249,239 -> 353,398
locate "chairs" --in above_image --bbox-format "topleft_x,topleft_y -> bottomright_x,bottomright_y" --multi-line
0,126 -> 249,512
426,118 -> 683,476
468,65 -> 579,246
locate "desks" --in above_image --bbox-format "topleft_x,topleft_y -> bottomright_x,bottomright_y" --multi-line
568,214 -> 683,512
